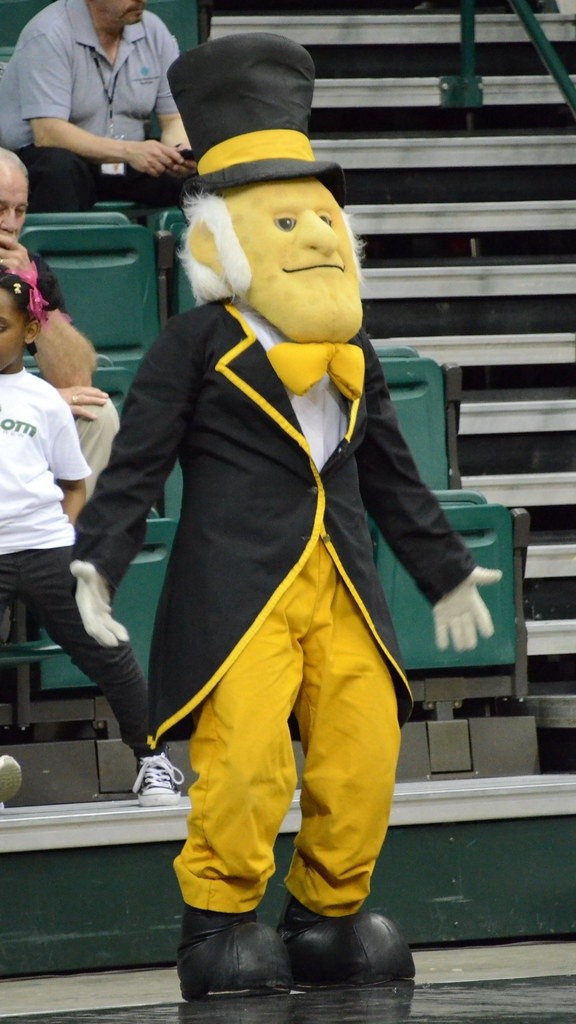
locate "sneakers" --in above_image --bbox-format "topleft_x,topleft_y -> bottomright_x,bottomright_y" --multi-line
0,755 -> 21,803
133,746 -> 185,807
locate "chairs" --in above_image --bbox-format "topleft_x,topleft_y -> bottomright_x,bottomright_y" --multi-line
375,349 -> 463,492
0,0 -> 198,807
371,490 -> 539,781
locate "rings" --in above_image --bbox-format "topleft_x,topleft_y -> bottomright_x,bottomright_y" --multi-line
0,259 -> 3,264
73,395 -> 79,405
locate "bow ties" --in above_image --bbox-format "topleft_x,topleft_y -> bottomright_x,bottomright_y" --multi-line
267,342 -> 366,402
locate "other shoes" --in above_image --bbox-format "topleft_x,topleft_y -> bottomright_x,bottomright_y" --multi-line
176,905 -> 292,1000
277,893 -> 416,989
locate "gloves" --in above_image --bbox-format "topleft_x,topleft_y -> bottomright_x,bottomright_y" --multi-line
69,560 -> 129,649
432,567 -> 503,652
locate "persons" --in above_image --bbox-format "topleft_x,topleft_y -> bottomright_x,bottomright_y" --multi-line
0,147 -> 120,505
0,266 -> 184,810
0,0 -> 197,214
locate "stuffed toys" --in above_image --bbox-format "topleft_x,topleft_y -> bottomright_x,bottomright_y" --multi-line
71,30 -> 503,996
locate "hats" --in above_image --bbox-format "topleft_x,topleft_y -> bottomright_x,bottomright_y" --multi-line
166,32 -> 347,210
